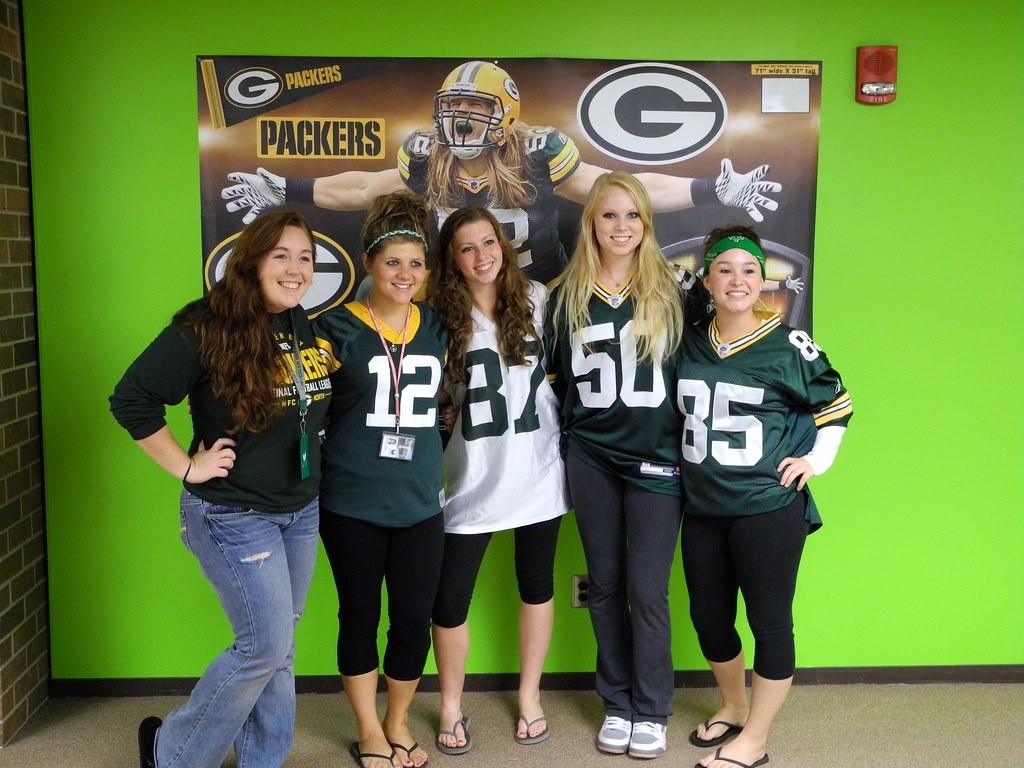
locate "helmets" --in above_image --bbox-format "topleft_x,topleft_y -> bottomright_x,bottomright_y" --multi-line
434,61 -> 520,146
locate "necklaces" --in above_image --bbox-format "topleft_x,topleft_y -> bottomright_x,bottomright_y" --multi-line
602,272 -> 628,287
379,321 -> 401,352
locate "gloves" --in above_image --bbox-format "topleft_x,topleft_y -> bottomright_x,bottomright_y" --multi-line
222,167 -> 314,225
785,276 -> 805,295
690,158 -> 783,223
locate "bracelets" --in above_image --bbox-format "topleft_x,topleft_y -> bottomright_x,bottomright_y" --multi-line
184,463 -> 192,481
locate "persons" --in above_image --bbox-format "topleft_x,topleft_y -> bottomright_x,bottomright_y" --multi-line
311,191 -> 460,768
443,170 -> 714,759
430,204 -> 571,755
219,60 -> 783,393
696,266 -> 805,312
108,210 -> 333,768
673,223 -> 854,768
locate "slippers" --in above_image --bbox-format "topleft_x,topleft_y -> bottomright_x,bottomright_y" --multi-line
695,746 -> 769,767
514,714 -> 550,745
389,739 -> 428,768
436,714 -> 473,754
689,716 -> 742,748
350,742 -> 393,767
138,717 -> 163,767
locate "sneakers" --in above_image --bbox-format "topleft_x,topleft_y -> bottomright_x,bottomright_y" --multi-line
597,715 -> 631,753
629,721 -> 667,758
